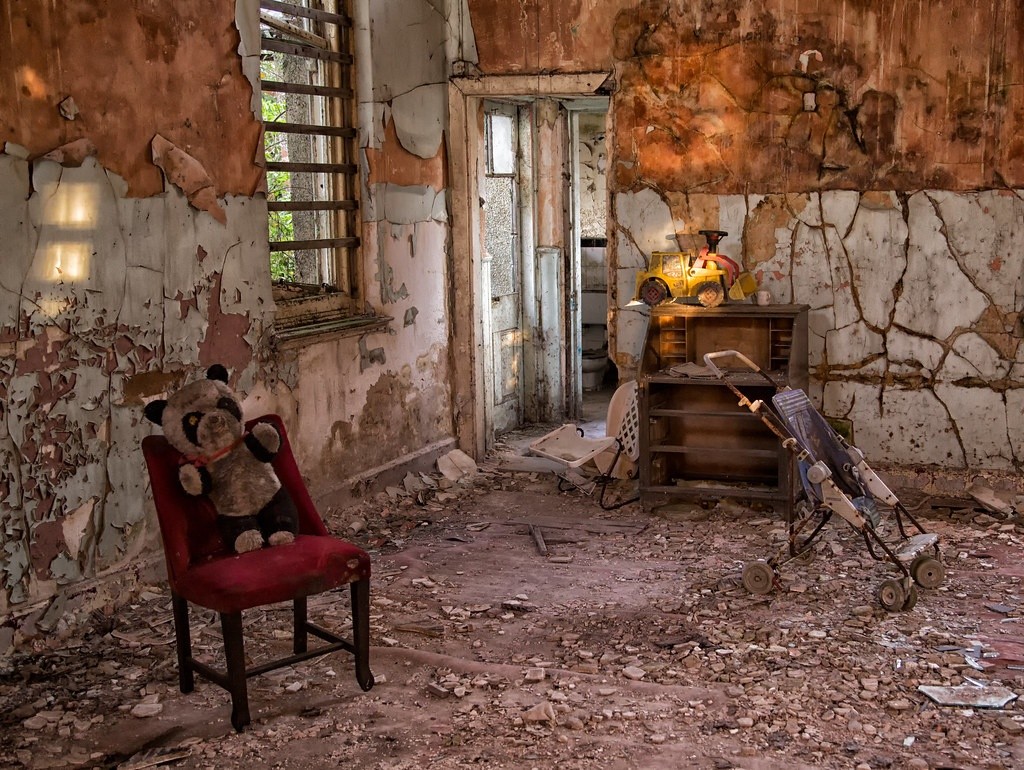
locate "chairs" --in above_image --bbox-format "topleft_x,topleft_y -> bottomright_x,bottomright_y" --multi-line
140,413 -> 372,734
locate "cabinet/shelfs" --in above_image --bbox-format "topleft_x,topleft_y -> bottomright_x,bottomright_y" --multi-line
635,301 -> 810,514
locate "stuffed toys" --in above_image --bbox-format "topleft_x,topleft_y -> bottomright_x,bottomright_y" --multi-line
144,364 -> 298,553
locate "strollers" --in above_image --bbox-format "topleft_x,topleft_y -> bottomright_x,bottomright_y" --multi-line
703,349 -> 945,613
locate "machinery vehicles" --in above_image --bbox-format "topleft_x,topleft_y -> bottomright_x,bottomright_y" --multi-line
629,230 -> 760,309
